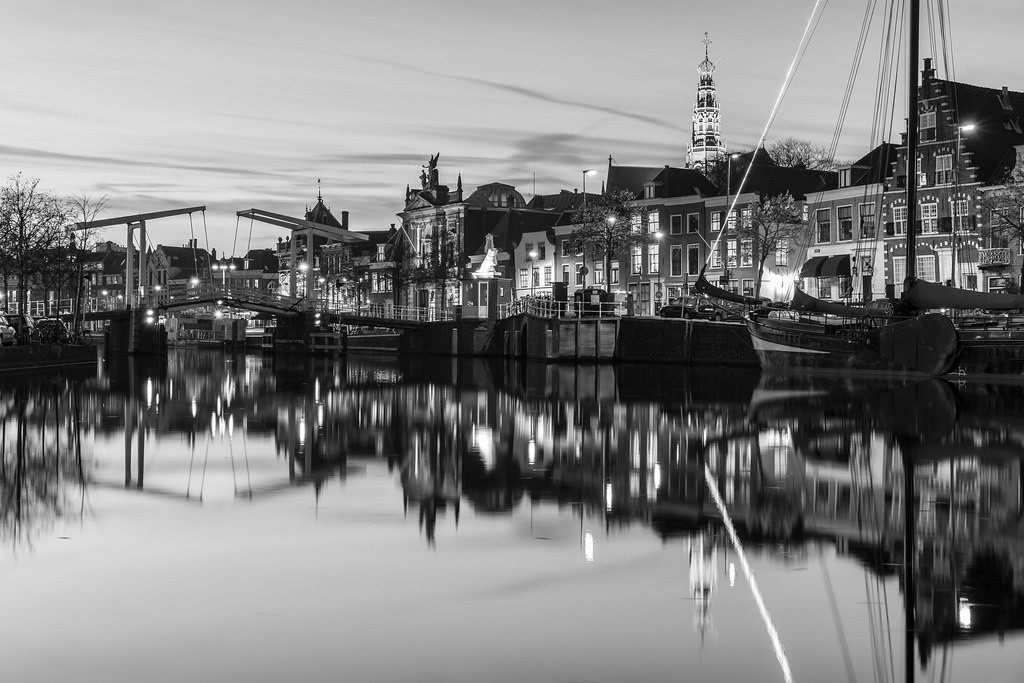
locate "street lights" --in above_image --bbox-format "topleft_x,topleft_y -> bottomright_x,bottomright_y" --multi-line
952,123 -> 976,285
581,168 -> 599,316
529,250 -> 537,297
654,231 -> 664,316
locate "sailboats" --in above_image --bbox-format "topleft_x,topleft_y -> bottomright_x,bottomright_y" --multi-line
661,0 -> 1023,387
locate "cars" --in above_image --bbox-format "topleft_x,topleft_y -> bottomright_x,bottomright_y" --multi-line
0,314 -> 43,346
37,318 -> 69,339
